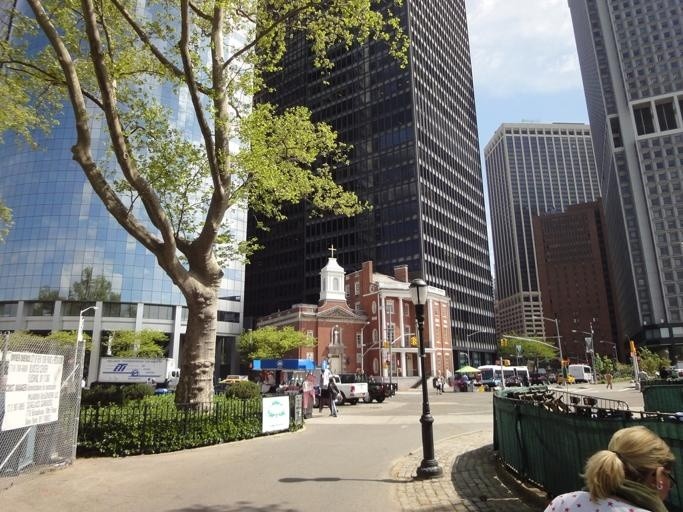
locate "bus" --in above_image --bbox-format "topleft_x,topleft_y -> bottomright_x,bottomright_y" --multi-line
476,364 -> 529,387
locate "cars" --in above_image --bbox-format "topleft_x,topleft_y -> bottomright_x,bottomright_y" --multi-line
215,368 -> 329,410
154,388 -> 172,394
529,367 -> 574,385
504,376 -> 523,387
660,360 -> 682,378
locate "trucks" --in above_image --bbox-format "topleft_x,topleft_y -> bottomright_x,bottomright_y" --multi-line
568,364 -> 592,383
96,355 -> 181,388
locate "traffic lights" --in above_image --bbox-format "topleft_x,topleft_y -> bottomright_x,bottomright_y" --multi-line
500,338 -> 507,347
410,336 -> 417,346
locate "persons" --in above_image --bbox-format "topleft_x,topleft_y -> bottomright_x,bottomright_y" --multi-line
660,366 -> 668,378
327,377 -> 339,418
315,367 -> 337,416
637,368 -> 650,393
461,374 -> 469,392
434,375 -> 443,395
605,370 -> 614,390
541,424 -> 676,511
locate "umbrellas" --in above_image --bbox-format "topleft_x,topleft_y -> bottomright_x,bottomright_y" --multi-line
455,366 -> 481,373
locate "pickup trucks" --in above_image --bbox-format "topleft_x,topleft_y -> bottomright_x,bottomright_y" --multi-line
320,371 -> 399,406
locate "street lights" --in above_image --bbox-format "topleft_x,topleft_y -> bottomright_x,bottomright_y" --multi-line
599,341 -> 618,367
572,329 -> 597,384
625,333 -> 630,343
467,331 -> 485,367
532,315 -> 565,387
72,305 -> 99,382
403,276 -> 444,478
